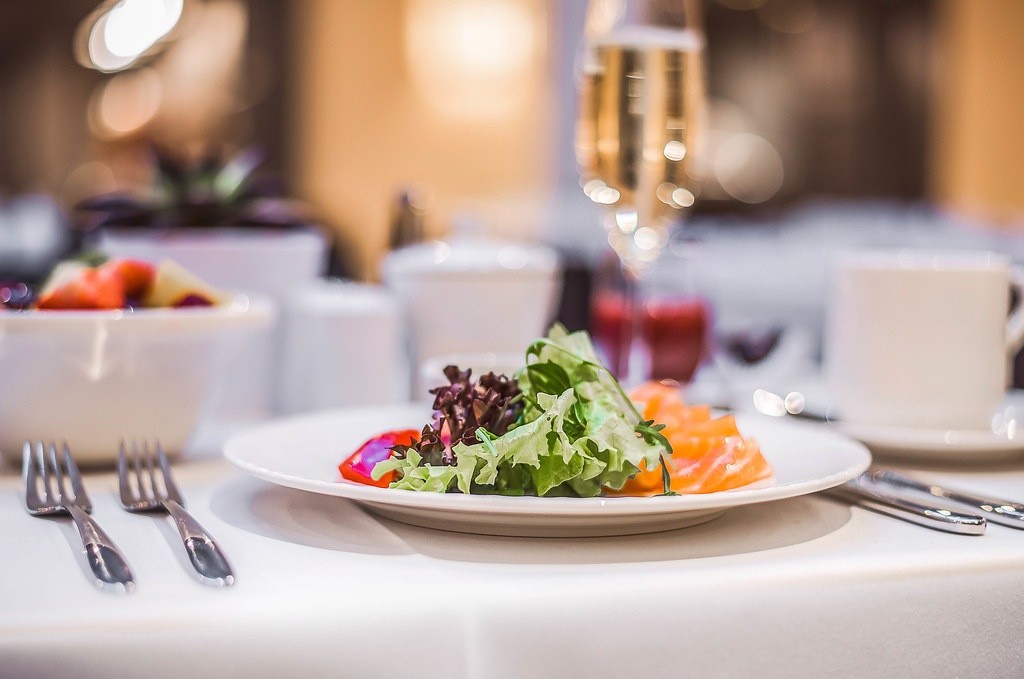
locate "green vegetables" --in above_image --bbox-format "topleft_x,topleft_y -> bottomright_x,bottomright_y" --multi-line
366,321 -> 680,502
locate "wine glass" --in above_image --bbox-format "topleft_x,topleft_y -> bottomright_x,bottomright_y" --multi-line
579,29 -> 708,384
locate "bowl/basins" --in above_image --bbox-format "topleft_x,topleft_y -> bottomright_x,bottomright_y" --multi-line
0,304 -> 279,467
102,227 -> 323,305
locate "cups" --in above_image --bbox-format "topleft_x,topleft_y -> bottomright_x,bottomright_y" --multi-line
279,289 -> 408,414
591,237 -> 712,388
824,250 -> 1024,429
379,239 -> 565,403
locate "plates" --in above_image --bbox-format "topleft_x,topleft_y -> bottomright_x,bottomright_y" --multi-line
835,390 -> 1024,466
222,402 -> 873,539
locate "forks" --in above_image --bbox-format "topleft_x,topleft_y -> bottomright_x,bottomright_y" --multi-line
118,435 -> 236,588
21,437 -> 135,594
